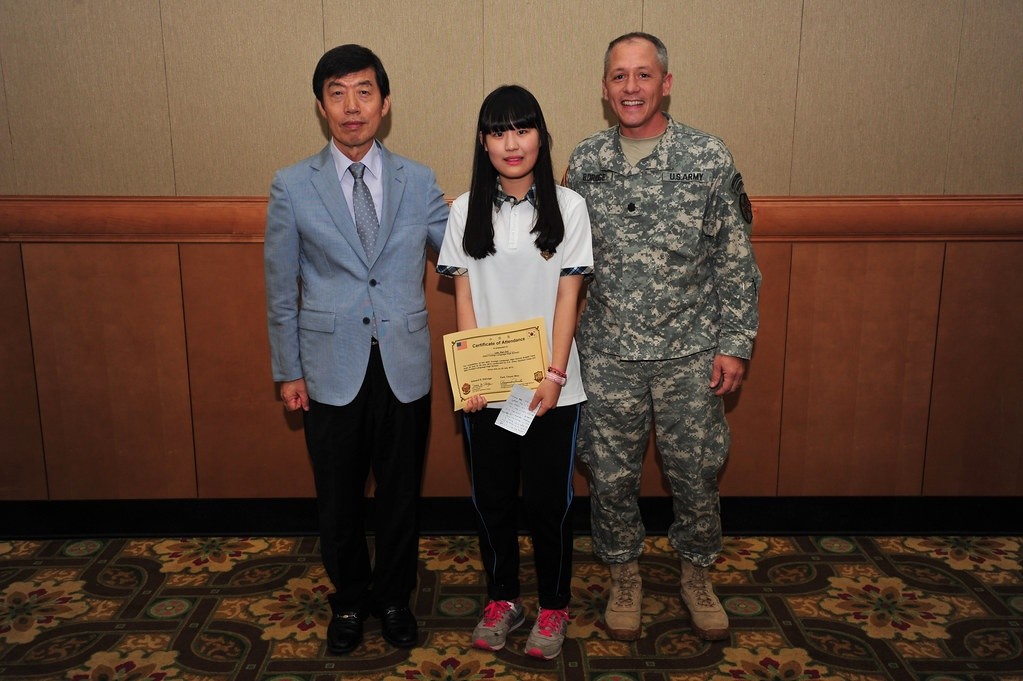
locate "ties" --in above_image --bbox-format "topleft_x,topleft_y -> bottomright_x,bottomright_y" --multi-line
348,163 -> 380,267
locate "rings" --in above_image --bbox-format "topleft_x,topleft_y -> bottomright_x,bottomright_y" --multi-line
737,384 -> 742,388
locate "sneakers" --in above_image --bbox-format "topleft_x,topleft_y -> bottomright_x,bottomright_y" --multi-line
523,607 -> 569,660
680,560 -> 729,639
473,597 -> 525,651
603,559 -> 642,641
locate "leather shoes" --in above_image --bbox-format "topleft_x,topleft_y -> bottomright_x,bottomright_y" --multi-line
326,608 -> 363,654
381,603 -> 419,647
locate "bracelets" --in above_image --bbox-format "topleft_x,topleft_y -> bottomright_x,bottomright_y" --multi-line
546,371 -> 565,386
548,366 -> 567,378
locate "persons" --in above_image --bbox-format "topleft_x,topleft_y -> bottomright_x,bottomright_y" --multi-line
264,44 -> 452,655
548,30 -> 762,637
436,84 -> 595,661
